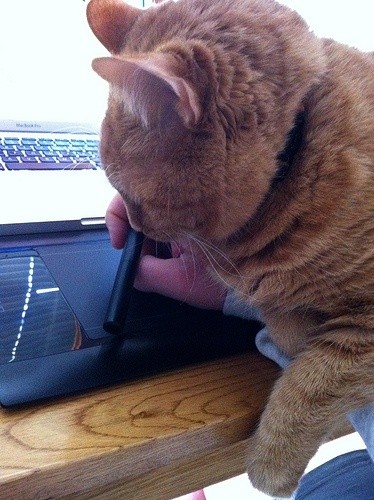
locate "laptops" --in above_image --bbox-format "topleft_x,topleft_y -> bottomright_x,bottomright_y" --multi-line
0,0 -> 153,238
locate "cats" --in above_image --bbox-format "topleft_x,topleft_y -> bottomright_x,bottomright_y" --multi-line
50,0 -> 374,500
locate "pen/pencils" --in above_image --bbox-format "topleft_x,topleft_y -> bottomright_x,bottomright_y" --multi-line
103,229 -> 143,334
